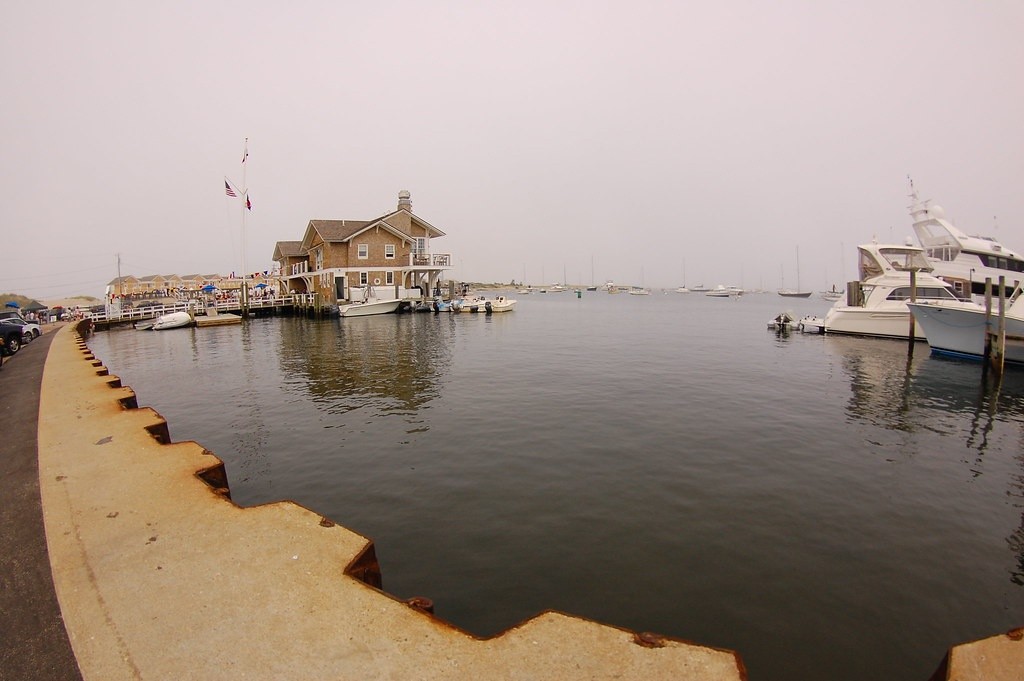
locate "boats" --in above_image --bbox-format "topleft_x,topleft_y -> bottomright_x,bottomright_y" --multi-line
689,284 -> 713,292
629,290 -> 649,295
823,175 -> 1024,339
778,289 -> 811,298
767,313 -> 798,330
906,277 -> 1024,365
517,290 -> 528,294
547,286 -> 568,292
705,285 -> 743,297
674,286 -> 689,293
586,287 -> 597,291
414,296 -> 517,312
824,287 -> 845,301
337,296 -> 402,316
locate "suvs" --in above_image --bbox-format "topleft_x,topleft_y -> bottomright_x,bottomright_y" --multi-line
50,306 -> 65,321
0,312 -> 38,324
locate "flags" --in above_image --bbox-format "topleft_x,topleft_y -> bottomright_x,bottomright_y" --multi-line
241,145 -> 250,164
246,195 -> 253,213
226,182 -> 237,198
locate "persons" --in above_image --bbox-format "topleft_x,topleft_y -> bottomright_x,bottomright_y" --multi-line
217,289 -> 276,301
24,310 -> 42,324
290,287 -> 308,294
66,306 -> 85,322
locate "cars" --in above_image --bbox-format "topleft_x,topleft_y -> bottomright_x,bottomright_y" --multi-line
0,318 -> 42,343
0,320 -> 24,354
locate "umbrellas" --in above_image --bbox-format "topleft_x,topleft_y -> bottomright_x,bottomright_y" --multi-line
257,282 -> 268,288
203,285 -> 216,290
23,300 -> 48,311
6,299 -> 21,310
